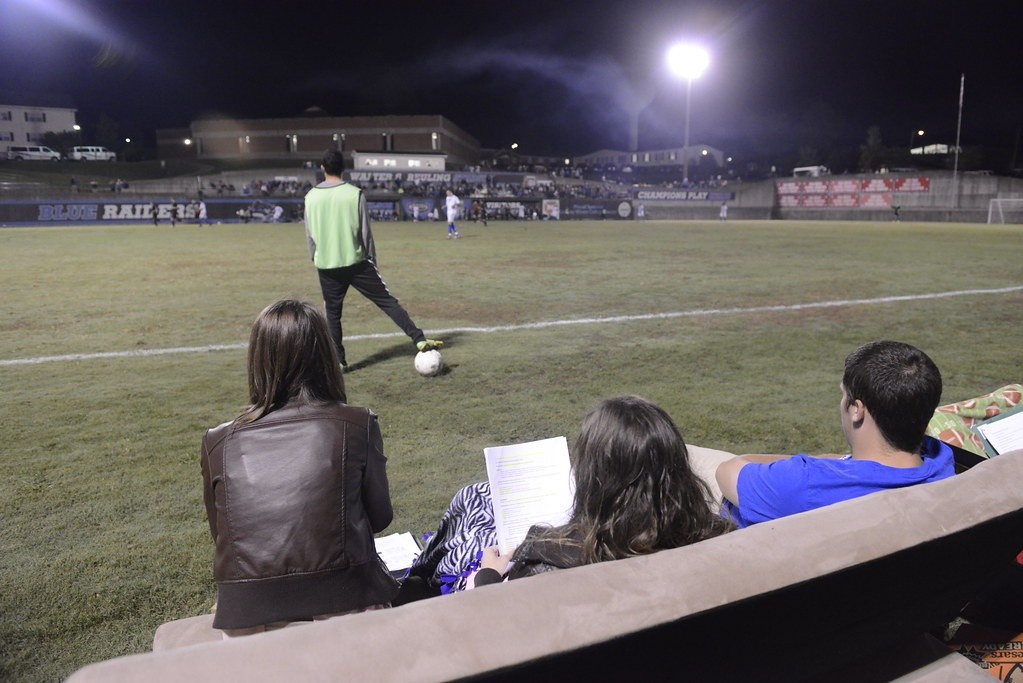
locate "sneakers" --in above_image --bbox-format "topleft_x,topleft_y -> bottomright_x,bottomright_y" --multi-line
416,338 -> 444,352
339,361 -> 347,371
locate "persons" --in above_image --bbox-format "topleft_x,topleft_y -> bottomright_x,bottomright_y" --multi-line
407,394 -> 744,595
198,299 -> 403,636
150,202 -> 161,227
197,199 -> 208,224
91,179 -> 98,192
678,339 -> 958,528
200,165 -> 728,222
167,202 -> 182,224
68,174 -> 80,194
302,149 -> 444,371
108,176 -> 128,193
445,190 -> 463,238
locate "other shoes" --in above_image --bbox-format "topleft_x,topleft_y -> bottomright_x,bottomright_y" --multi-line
446,234 -> 452,239
454,232 -> 459,239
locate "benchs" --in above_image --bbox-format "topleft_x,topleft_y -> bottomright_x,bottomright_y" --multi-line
64,446 -> 1023,683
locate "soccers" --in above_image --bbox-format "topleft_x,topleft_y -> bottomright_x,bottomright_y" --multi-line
413,349 -> 444,377
